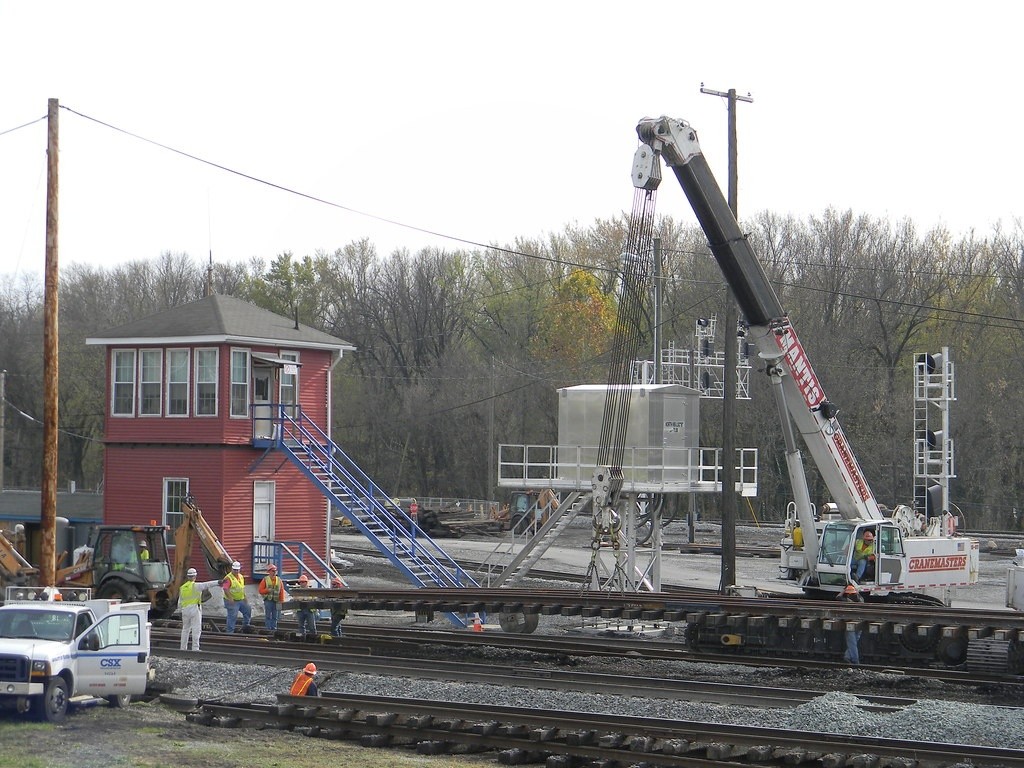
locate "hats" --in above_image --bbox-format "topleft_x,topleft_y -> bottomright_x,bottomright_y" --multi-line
138,541 -> 146,548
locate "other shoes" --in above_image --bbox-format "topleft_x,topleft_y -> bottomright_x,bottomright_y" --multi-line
243,624 -> 255,629
851,573 -> 860,583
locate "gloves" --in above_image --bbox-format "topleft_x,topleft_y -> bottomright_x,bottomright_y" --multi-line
217,578 -> 228,585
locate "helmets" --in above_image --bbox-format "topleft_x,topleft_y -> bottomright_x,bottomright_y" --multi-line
298,575 -> 309,584
232,561 -> 242,569
332,578 -> 342,585
302,662 -> 317,675
843,586 -> 858,595
187,567 -> 198,577
265,563 -> 278,573
863,531 -> 875,542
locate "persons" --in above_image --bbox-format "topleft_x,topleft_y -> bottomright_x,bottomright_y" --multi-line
839,532 -> 875,584
130,540 -> 150,562
175,567 -> 228,651
259,564 -> 284,631
222,561 -> 252,634
329,578 -> 349,646
294,575 -> 318,638
289,662 -> 321,715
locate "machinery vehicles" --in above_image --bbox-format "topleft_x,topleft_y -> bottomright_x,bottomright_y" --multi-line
508,490 -> 563,537
580,114 -> 978,657
0,491 -> 238,620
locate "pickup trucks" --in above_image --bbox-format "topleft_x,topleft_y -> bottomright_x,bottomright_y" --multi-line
0,585 -> 153,725
